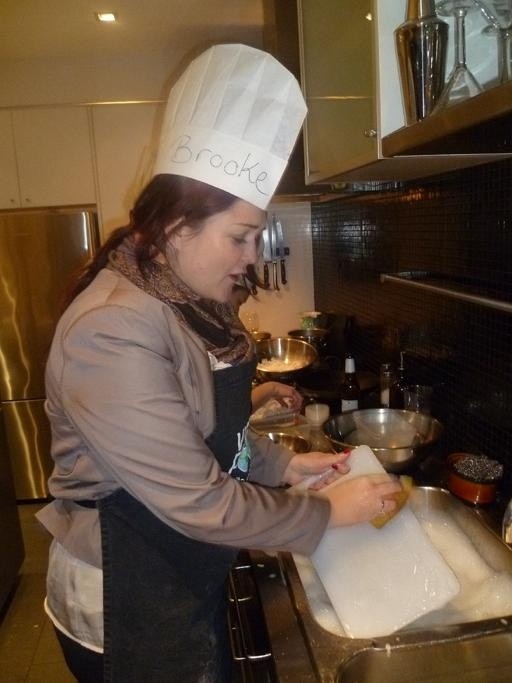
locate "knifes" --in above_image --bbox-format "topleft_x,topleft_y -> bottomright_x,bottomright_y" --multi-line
263,214 -> 287,292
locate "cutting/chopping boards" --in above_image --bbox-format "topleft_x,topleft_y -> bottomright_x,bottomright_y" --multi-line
286,445 -> 461,638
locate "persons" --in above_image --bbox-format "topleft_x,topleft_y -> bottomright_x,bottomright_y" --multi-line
230,283 -> 304,413
34,41 -> 404,683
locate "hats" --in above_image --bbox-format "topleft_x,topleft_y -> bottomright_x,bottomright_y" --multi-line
152,42 -> 309,211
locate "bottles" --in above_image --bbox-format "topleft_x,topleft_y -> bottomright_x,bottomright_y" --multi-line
336,352 -> 361,414
389,351 -> 413,409
394,0 -> 449,126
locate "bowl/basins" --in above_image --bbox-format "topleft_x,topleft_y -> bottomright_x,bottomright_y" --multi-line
320,407 -> 444,474
256,430 -> 312,454
249,331 -> 271,341
286,329 -> 331,351
254,336 -> 318,383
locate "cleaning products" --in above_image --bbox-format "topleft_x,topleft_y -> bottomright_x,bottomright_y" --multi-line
299,312 -> 322,329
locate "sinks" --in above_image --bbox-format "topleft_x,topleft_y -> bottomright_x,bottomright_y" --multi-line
291,483 -> 512,652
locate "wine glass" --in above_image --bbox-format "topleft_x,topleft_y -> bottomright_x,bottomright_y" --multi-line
429,0 -> 483,116
472,1 -> 511,84
